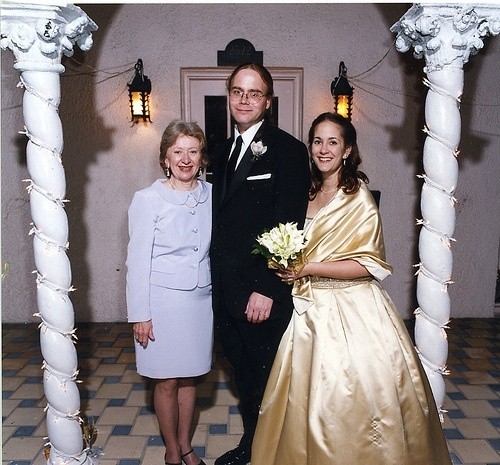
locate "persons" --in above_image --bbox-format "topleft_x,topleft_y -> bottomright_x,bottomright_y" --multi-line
125,120 -> 216,465
251,112 -> 452,464
211,62 -> 312,465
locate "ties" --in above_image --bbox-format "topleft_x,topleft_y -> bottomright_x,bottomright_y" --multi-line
224,135 -> 243,191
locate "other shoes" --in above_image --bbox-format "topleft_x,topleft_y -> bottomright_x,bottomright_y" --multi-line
181,449 -> 206,465
164,451 -> 182,465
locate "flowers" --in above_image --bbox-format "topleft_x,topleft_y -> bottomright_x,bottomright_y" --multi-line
250,221 -> 308,269
250,141 -> 268,164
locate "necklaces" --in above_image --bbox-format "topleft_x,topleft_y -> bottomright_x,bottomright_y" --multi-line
320,187 -> 338,195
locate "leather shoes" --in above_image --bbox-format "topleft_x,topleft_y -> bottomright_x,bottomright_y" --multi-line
214,442 -> 251,465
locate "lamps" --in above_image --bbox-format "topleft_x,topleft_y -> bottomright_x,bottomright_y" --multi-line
127,58 -> 153,123
331,61 -> 355,121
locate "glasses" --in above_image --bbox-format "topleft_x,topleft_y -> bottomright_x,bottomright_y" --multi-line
229,88 -> 269,103
